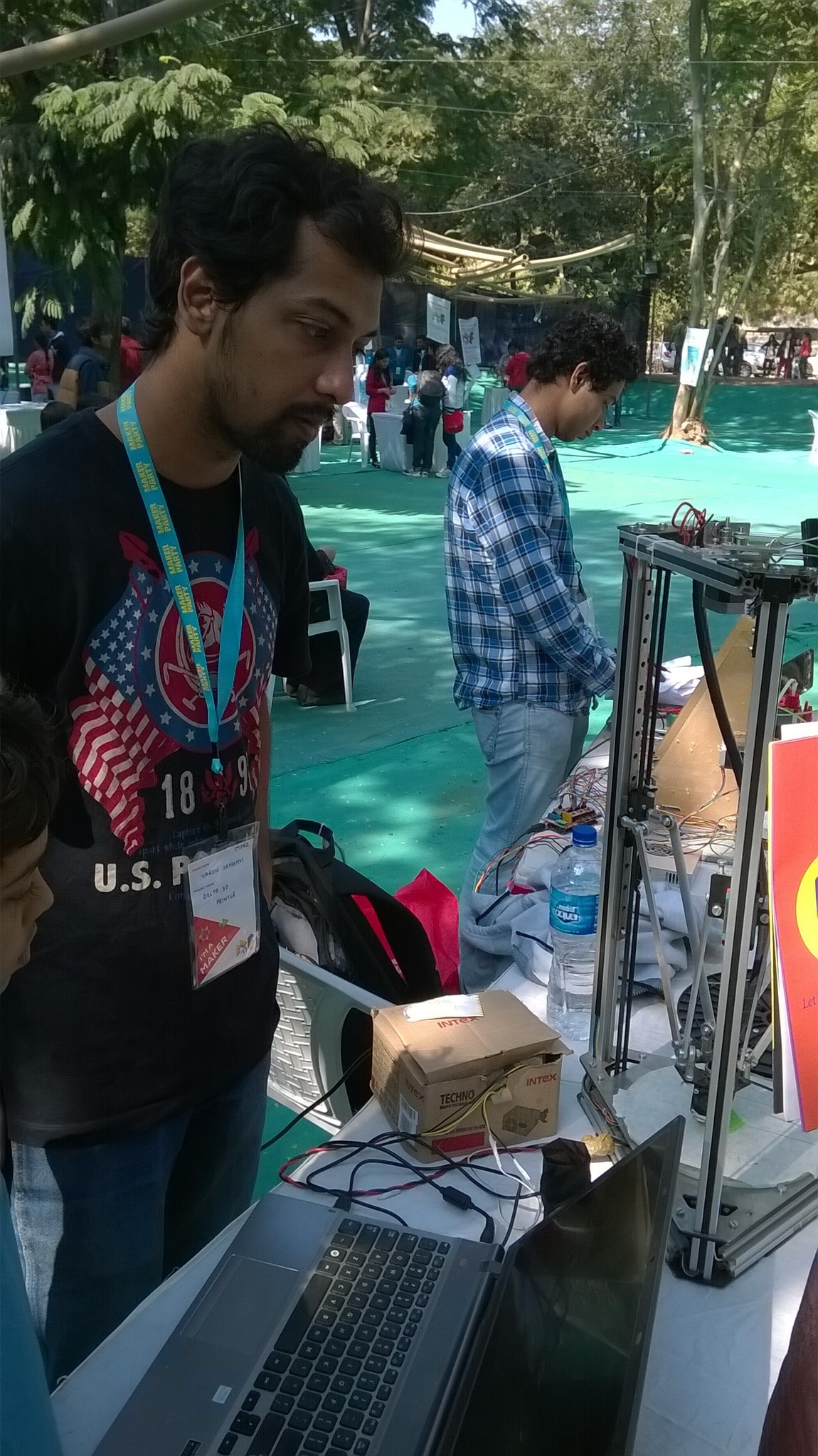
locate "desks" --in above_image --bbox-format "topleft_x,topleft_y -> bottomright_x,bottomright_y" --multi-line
371,408 -> 473,472
0,403 -> 49,460
482,386 -> 512,426
49,704 -> 818,1456
286,425 -> 321,473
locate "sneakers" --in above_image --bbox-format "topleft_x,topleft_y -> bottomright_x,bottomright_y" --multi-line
434,467 -> 452,478
402,469 -> 421,477
421,471 -> 428,478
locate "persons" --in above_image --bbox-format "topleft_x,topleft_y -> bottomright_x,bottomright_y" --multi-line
0,117 -> 416,1395
285,534 -> 370,708
0,685 -> 64,1456
445,314 -> 665,994
24,313 -> 811,479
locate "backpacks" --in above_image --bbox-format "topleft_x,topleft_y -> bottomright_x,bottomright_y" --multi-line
263,818 -> 446,1131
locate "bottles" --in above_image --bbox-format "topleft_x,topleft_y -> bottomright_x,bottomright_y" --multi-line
547,825 -> 603,1041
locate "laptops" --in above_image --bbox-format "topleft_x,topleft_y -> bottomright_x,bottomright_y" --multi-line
93,1112 -> 688,1456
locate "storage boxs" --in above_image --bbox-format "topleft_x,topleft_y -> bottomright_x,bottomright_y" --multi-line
363,977 -> 568,1166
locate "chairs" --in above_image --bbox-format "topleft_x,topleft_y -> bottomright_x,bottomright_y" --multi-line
266,576 -> 357,723
0,389 -> 20,406
463,378 -> 475,411
341,400 -> 378,468
389,385 -> 411,410
